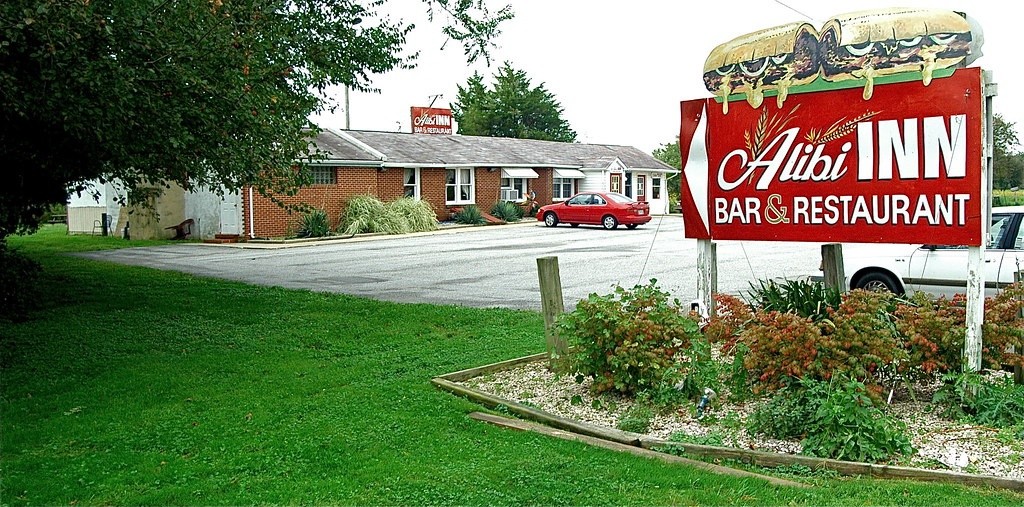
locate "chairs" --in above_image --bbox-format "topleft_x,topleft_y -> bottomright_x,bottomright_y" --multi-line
594,199 -> 599,204
91,215 -> 114,238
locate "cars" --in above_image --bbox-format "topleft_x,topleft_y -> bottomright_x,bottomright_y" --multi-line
535,190 -> 652,231
842,205 -> 1024,307
